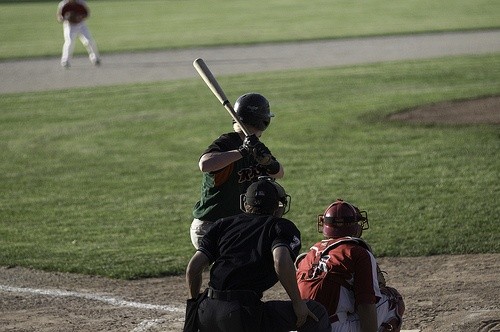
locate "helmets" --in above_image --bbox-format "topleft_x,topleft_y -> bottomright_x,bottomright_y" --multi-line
233,93 -> 275,120
317,201 -> 363,239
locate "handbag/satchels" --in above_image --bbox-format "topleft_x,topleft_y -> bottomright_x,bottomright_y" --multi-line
183,289 -> 208,332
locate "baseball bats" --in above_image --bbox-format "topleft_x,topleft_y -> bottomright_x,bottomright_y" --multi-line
192,57 -> 250,137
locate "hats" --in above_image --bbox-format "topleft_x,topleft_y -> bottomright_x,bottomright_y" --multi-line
246,181 -> 279,208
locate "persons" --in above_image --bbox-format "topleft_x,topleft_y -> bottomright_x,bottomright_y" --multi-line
56,0 -> 101,70
294,198 -> 406,332
183,175 -> 329,332
189,92 -> 284,251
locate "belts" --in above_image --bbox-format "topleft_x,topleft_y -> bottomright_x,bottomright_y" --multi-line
207,289 -> 264,302
329,311 -> 351,324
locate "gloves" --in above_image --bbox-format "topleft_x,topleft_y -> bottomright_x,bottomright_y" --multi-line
250,143 -> 280,175
238,133 -> 260,157
